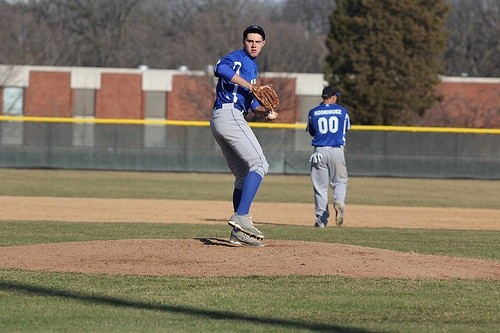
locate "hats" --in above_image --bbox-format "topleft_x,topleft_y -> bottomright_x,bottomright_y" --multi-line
321,86 -> 341,99
242,24 -> 265,40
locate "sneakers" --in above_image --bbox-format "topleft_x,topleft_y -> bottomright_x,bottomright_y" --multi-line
229,228 -> 264,247
228,212 -> 264,240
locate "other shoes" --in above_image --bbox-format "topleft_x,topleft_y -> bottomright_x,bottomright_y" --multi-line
334,201 -> 344,226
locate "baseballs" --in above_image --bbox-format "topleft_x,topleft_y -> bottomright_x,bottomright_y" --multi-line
266,110 -> 278,120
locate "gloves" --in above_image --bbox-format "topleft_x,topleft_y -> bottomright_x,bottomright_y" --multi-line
309,152 -> 323,164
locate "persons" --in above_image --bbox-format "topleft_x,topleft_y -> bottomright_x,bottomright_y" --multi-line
306,87 -> 350,228
209,25 -> 279,247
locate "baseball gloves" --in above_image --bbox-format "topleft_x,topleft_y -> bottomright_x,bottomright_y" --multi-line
252,85 -> 280,110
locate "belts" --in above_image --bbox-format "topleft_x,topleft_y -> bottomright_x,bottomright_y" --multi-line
215,103 -> 242,112
317,145 -> 340,148
